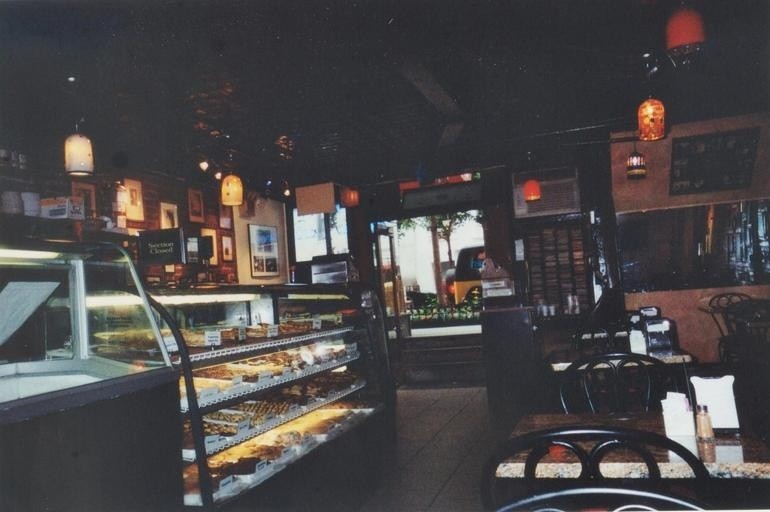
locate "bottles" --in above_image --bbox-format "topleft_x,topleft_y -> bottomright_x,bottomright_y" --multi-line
696,406 -> 715,439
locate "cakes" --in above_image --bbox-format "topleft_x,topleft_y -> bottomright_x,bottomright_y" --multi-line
182,407 -> 355,493
90,314 -> 341,359
178,343 -> 348,403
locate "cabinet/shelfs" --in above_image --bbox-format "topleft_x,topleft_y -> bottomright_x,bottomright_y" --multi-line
42,280 -> 396,512
0,240 -> 173,403
525,220 -> 595,315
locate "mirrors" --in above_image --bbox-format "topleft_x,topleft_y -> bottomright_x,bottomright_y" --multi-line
615,197 -> 769,292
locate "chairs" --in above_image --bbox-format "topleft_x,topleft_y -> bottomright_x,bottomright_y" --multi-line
576,313 -> 652,353
483,425 -> 713,511
710,293 -> 753,375
558,353 -> 680,414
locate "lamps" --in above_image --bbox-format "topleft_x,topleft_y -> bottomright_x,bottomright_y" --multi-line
221,133 -> 243,206
637,70 -> 665,141
524,143 -> 541,201
626,128 -> 648,179
64,74 -> 95,176
339,185 -> 360,208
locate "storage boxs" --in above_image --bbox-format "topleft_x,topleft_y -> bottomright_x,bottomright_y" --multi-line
41,197 -> 85,221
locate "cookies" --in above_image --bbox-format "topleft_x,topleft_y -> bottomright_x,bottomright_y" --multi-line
183,371 -> 354,452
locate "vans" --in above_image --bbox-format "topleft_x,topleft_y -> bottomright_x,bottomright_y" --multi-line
454,249 -> 488,308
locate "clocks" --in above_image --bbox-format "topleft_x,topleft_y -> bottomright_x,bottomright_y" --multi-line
670,128 -> 757,196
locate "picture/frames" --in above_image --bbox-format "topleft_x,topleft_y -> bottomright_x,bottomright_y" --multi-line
247,223 -> 281,277
69,174 -> 234,266
